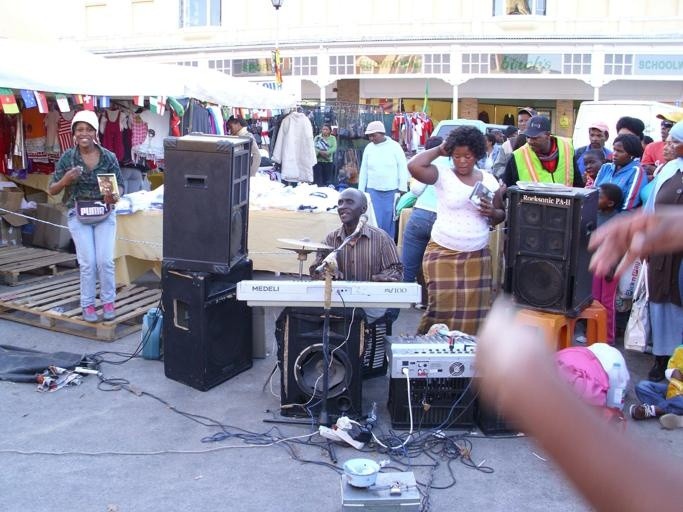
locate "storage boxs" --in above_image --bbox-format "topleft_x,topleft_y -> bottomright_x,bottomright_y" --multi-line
26,205 -> 74,252
0,212 -> 23,248
0,173 -> 25,214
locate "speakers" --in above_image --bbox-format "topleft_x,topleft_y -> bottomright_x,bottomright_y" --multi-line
161,259 -> 254,392
280,305 -> 366,423
161,129 -> 253,275
504,183 -> 601,319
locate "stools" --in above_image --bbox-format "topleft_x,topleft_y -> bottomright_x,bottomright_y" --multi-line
569,299 -> 608,344
354,310 -> 392,381
510,308 -> 571,353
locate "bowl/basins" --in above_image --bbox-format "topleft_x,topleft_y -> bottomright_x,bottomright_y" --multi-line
344,459 -> 380,488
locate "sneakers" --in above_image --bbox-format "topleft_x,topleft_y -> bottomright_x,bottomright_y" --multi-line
103,303 -> 116,321
650,360 -> 667,381
659,414 -> 683,429
82,304 -> 98,322
629,404 -> 656,420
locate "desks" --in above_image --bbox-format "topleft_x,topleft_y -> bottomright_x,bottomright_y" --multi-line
95,183 -> 379,289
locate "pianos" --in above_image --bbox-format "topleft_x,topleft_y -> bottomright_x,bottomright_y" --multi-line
236,279 -> 423,308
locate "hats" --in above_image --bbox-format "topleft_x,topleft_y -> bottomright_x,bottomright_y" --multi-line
70,110 -> 99,133
364,121 -> 386,136
492,106 -> 552,136
665,120 -> 683,143
588,121 -> 609,134
656,108 -> 683,123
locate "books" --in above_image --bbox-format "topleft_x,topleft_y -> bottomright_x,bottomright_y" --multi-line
96,173 -> 120,196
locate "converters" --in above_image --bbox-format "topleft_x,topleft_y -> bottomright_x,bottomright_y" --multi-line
348,425 -> 372,441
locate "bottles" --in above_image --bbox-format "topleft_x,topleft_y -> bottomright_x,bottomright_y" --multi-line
606,363 -> 624,409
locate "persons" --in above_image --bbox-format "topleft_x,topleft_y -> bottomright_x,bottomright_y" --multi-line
309,187 -> 404,283
49,108 -> 125,324
228,114 -> 261,179
470,204 -> 683,512
357,118 -> 409,242
313,123 -> 338,187
400,106 -> 682,430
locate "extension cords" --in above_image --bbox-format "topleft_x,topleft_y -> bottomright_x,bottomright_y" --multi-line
332,427 -> 365,450
75,366 -> 99,375
318,425 -> 351,444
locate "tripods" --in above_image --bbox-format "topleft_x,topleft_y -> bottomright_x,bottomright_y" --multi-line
260,278 -> 369,464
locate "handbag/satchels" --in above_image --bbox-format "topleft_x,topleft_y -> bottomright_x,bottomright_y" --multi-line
396,190 -> 418,216
624,260 -> 650,352
554,346 -> 609,408
75,199 -> 112,223
315,137 -> 332,162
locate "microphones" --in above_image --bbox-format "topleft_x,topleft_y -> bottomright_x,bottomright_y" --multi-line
354,213 -> 369,237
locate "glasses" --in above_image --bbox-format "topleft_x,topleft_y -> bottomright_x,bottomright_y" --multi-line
661,121 -> 673,128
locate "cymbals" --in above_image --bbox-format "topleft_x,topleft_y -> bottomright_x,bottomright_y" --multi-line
277,238 -> 333,249
